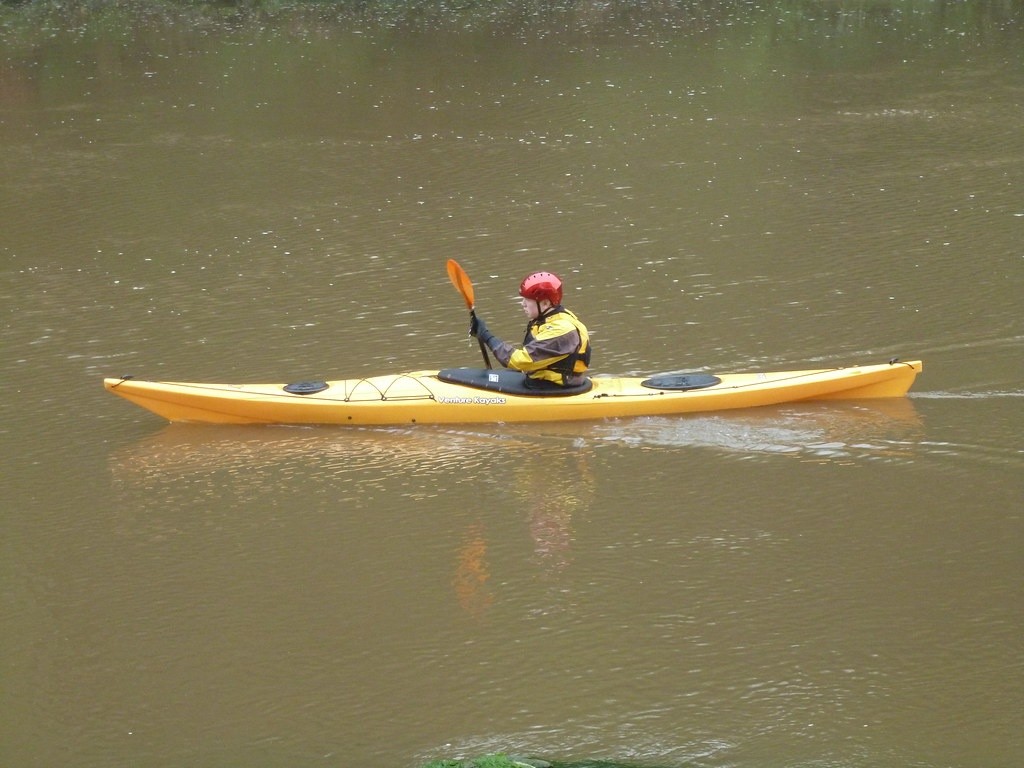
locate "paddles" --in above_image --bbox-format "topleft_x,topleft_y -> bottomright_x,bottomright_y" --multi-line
446,259 -> 493,370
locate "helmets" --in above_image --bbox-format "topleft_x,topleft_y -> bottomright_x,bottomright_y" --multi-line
518,272 -> 563,305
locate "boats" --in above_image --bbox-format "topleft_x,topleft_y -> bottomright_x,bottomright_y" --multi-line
103,359 -> 932,429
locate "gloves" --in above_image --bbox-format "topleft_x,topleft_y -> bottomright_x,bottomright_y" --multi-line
469,316 -> 488,339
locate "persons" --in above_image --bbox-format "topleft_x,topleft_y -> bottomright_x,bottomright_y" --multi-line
469,272 -> 591,388
